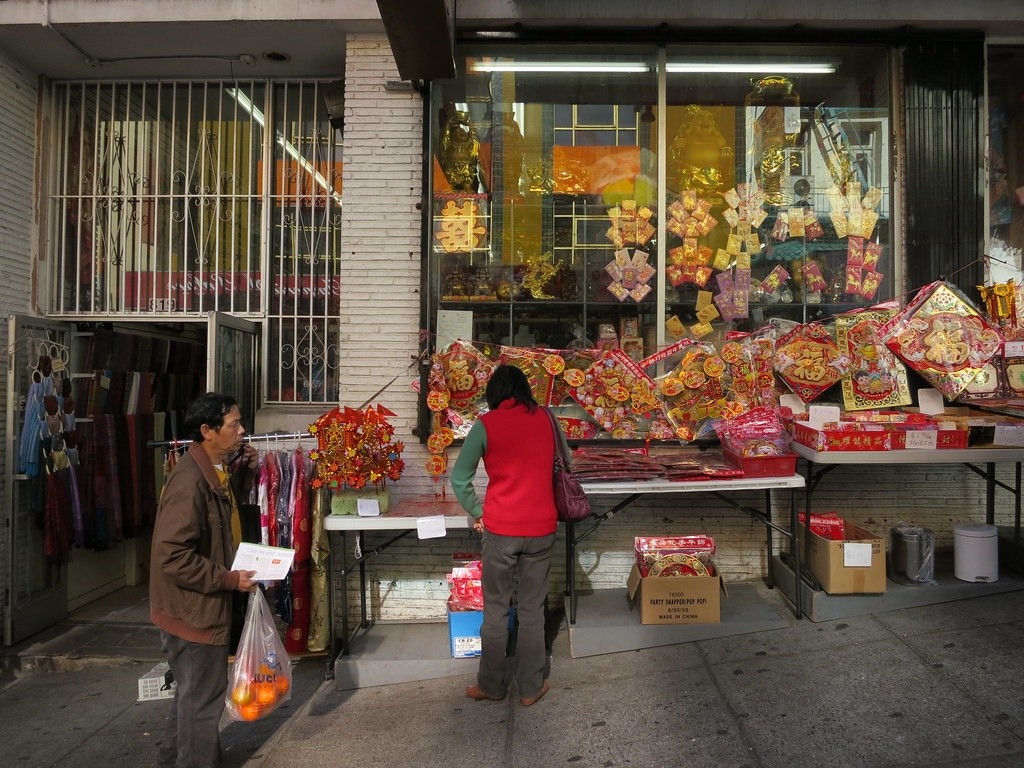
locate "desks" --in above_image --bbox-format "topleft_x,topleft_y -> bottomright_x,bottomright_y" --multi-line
322,496 -> 548,657
790,442 -> 1024,592
566,472 -> 806,624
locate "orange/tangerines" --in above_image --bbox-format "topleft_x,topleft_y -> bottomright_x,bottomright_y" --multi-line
231,662 -> 288,722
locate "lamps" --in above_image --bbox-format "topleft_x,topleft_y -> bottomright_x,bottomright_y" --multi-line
666,57 -> 841,74
466,54 -> 660,73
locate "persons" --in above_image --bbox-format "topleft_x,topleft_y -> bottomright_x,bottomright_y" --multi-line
742,88 -> 800,177
442,116 -> 480,194
671,110 -> 733,194
149,392 -> 258,768
451,364 -> 570,706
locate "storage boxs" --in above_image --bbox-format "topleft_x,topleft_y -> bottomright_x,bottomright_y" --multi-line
136,662 -> 177,702
720,443 -> 799,478
446,593 -> 514,659
626,558 -> 729,625
777,407 -> 1023,452
793,518 -> 886,595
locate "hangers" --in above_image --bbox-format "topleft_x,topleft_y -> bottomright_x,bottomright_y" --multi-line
160,431 -> 300,464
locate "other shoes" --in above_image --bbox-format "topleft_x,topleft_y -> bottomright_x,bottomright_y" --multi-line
465,684 -> 505,700
519,681 -> 549,706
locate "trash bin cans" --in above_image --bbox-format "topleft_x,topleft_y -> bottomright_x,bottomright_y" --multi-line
889,522 -> 935,586
953,525 -> 1000,585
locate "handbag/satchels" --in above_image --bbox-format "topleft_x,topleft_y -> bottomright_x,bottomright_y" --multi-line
540,406 -> 592,522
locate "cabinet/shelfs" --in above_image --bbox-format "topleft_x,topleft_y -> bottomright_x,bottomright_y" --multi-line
440,187 -> 890,372
